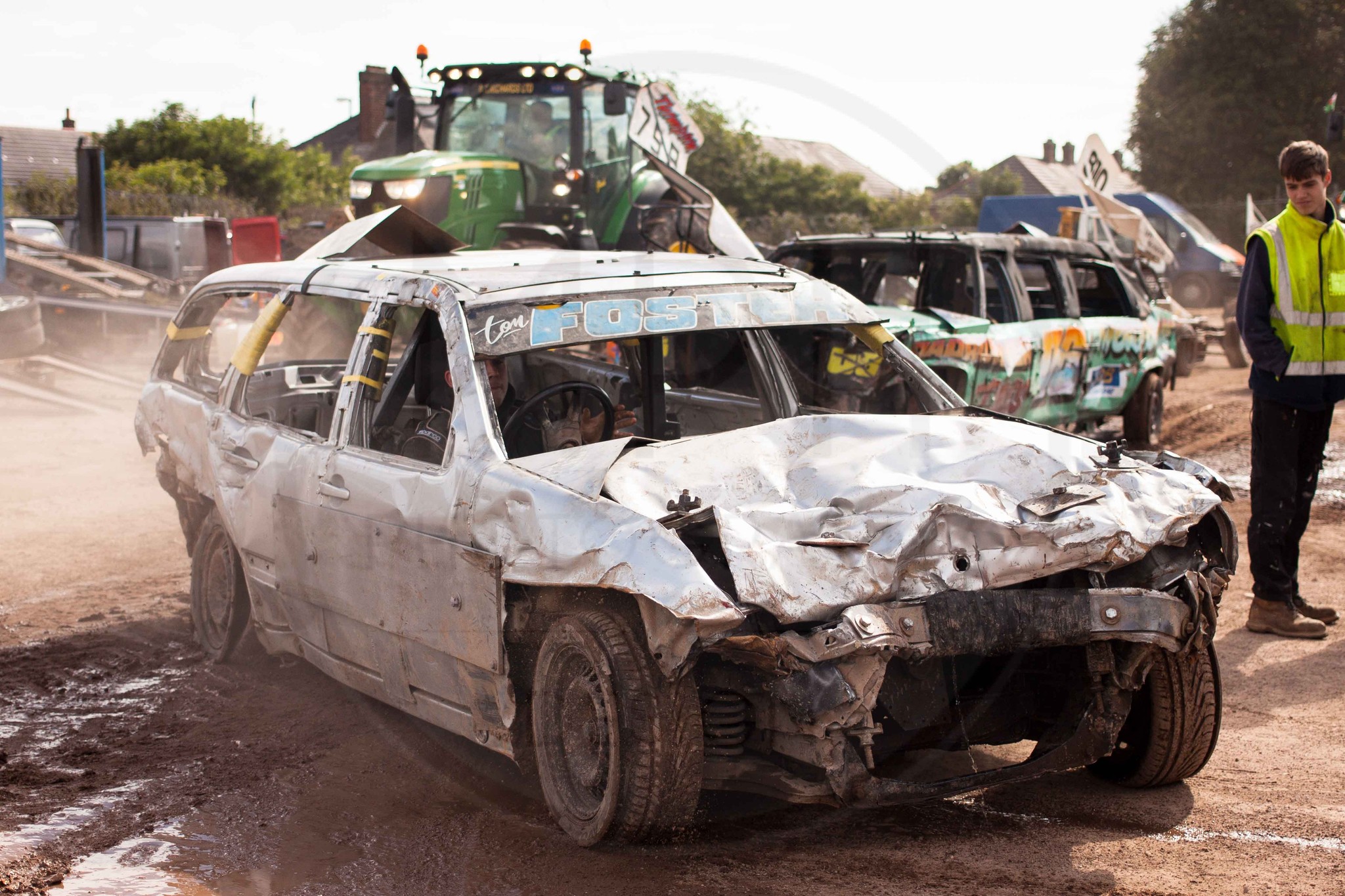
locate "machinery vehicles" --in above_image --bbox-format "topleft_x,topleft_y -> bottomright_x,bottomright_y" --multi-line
348,40 -> 712,255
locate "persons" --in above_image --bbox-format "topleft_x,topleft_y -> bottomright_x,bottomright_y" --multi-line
1236,141 -> 1345,639
400,354 -> 636,465
517,101 -> 570,171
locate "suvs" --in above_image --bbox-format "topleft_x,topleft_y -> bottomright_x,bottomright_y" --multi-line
134,206 -> 1237,855
762,223 -> 1177,452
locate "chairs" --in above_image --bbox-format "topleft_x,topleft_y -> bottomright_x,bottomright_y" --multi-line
826,263 -> 878,306
391,339 -> 455,432
922,258 -> 998,324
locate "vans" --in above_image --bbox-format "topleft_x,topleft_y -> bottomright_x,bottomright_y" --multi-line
978,192 -> 1248,368
4,217 -> 69,266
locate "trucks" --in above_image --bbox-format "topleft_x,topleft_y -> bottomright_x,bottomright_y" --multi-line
30,210 -> 281,296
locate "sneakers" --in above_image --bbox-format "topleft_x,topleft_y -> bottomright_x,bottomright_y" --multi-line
1246,595 -> 1328,639
1290,593 -> 1338,624
